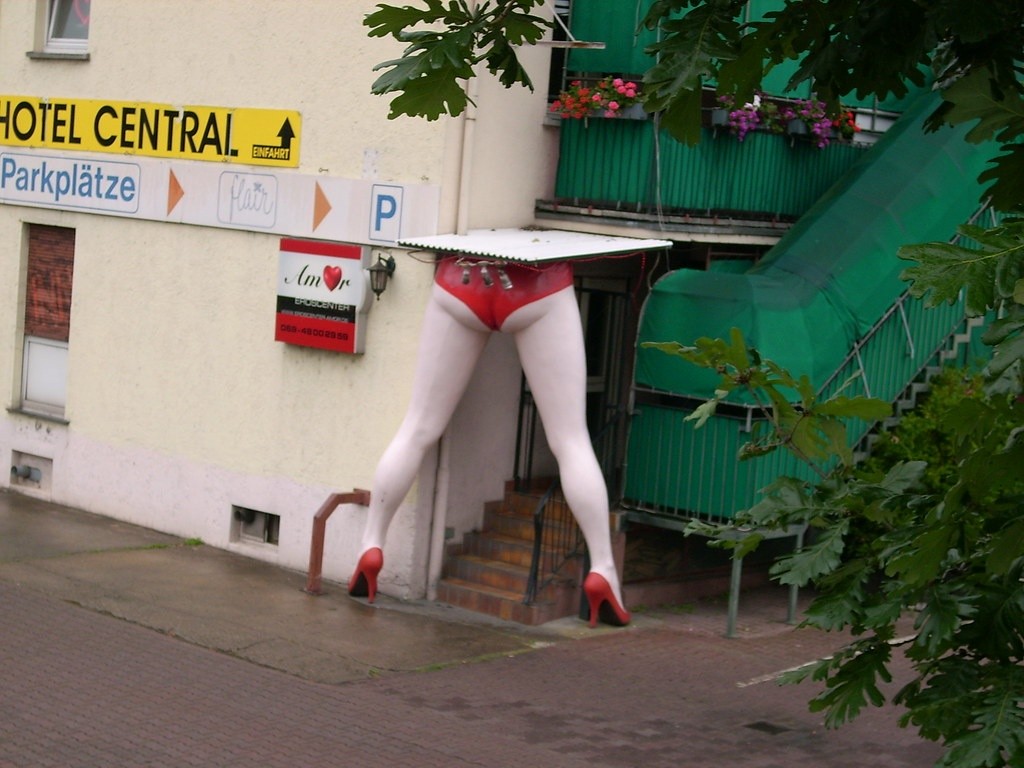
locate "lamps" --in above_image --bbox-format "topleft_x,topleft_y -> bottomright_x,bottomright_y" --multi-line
365,253 -> 396,301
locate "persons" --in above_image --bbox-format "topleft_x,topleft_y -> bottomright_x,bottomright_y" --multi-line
347,256 -> 631,628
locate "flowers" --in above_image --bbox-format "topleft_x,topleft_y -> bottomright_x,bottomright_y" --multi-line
714,91 -> 862,147
549,74 -> 636,128
